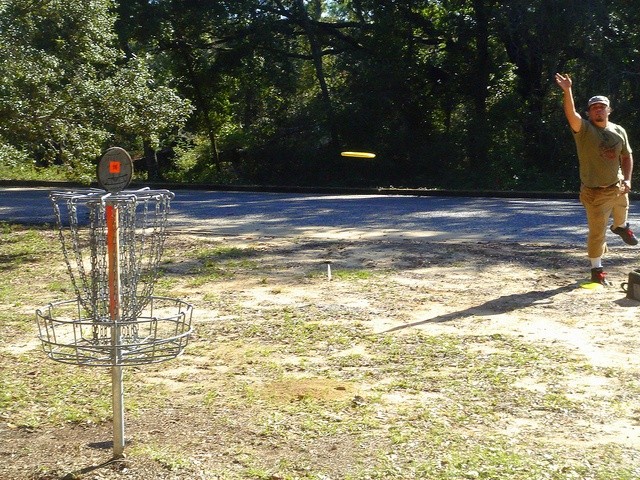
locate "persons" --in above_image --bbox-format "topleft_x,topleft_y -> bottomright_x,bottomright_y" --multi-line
555,72 -> 639,289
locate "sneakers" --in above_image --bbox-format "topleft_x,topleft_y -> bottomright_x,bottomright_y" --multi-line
610,223 -> 640,245
591,268 -> 613,287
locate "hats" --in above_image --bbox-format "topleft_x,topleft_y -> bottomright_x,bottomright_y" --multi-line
587,96 -> 609,107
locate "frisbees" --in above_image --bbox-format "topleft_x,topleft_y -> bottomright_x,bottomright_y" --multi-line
341,152 -> 376,158
